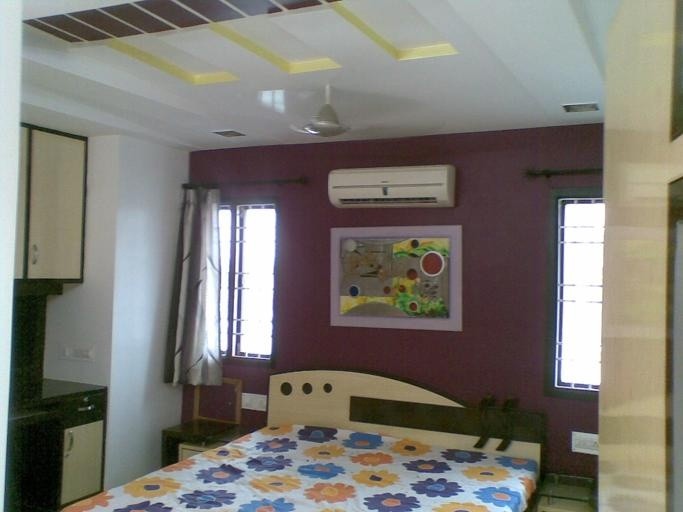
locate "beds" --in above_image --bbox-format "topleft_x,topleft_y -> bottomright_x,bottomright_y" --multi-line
57,368 -> 550,511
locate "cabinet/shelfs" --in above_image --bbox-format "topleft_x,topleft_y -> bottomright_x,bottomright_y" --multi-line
19,121 -> 87,285
26,390 -> 104,510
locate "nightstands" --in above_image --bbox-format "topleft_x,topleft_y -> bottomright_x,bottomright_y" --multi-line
160,420 -> 256,468
534,472 -> 597,512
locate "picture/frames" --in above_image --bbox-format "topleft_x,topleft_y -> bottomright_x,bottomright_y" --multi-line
329,225 -> 464,331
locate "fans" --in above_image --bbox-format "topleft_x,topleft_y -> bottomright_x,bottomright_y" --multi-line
199,79 -> 453,139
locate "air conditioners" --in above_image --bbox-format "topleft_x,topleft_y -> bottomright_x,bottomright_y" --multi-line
327,165 -> 457,208
